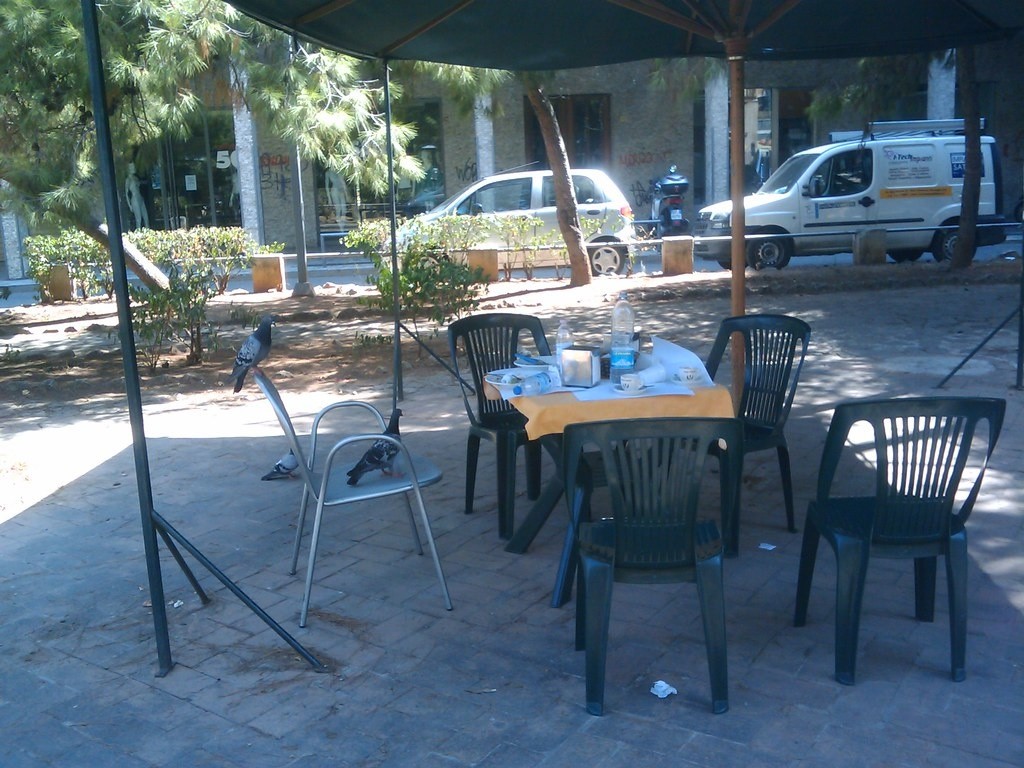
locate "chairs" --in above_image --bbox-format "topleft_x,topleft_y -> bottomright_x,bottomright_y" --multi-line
448,313 -> 592,539
551,417 -> 744,716
253,367 -> 453,628
790,395 -> 1006,685
656,313 -> 811,557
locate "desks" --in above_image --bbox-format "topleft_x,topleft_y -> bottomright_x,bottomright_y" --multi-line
483,345 -> 741,608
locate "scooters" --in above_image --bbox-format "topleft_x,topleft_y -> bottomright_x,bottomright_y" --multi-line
646,164 -> 690,237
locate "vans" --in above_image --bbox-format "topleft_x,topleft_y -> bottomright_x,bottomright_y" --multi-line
695,116 -> 1006,272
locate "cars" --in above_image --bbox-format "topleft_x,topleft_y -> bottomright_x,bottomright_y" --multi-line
400,179 -> 446,219
379,168 -> 636,277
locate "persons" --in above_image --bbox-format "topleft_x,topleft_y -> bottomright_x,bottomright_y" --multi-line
228,173 -> 241,208
125,162 -> 150,231
324,167 -> 352,234
394,147 -> 445,219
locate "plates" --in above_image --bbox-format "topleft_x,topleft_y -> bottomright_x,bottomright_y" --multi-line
671,377 -> 704,385
514,355 -> 561,370
613,384 -> 649,394
484,373 -> 526,387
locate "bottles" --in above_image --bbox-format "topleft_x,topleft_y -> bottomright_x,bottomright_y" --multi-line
609,292 -> 637,389
557,321 -> 574,377
513,371 -> 556,396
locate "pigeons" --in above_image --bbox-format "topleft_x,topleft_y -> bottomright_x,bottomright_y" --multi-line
346,409 -> 404,485
261,449 -> 299,480
225,313 -> 276,393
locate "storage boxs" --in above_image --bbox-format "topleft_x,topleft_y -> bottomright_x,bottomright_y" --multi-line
562,346 -> 602,387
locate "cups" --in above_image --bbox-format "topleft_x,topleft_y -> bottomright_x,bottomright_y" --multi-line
673,367 -> 702,381
639,333 -> 655,355
620,374 -> 645,390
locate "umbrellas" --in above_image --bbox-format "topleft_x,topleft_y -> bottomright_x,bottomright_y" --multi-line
227,0 -> 1023,429
